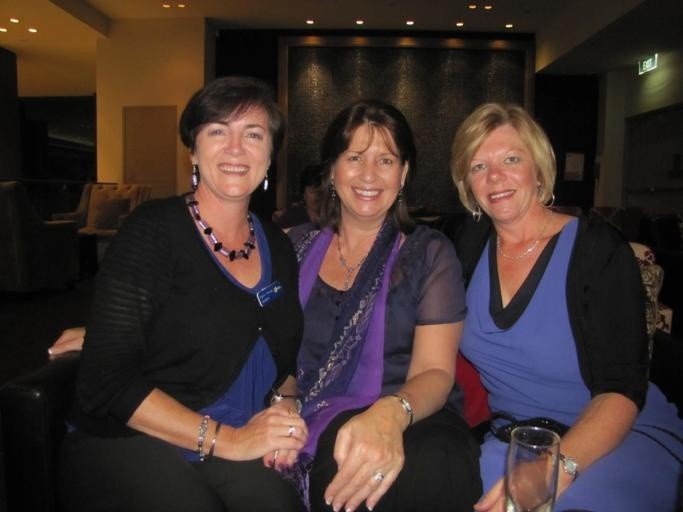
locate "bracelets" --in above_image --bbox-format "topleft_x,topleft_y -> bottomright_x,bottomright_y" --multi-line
197,415 -> 221,462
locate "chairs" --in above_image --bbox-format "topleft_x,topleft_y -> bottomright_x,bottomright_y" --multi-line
419,203 -> 682,316
0,172 -> 154,299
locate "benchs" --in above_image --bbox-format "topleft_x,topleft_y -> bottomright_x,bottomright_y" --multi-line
0,323 -> 681,511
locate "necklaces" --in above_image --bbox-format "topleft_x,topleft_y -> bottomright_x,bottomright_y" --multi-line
185,192 -> 256,261
336,225 -> 379,290
496,213 -> 554,259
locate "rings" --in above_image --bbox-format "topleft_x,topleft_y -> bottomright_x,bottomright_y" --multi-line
374,471 -> 384,480
288,408 -> 297,415
289,426 -> 295,437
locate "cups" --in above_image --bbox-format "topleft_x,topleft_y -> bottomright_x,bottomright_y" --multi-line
502,427 -> 563,511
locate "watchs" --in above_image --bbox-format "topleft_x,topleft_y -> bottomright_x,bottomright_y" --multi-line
388,394 -> 413,427
548,450 -> 579,482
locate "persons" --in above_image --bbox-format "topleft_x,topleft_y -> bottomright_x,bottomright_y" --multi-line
275,170 -> 322,230
48,98 -> 483,512
449,100 -> 683,512
58,75 -> 309,512
